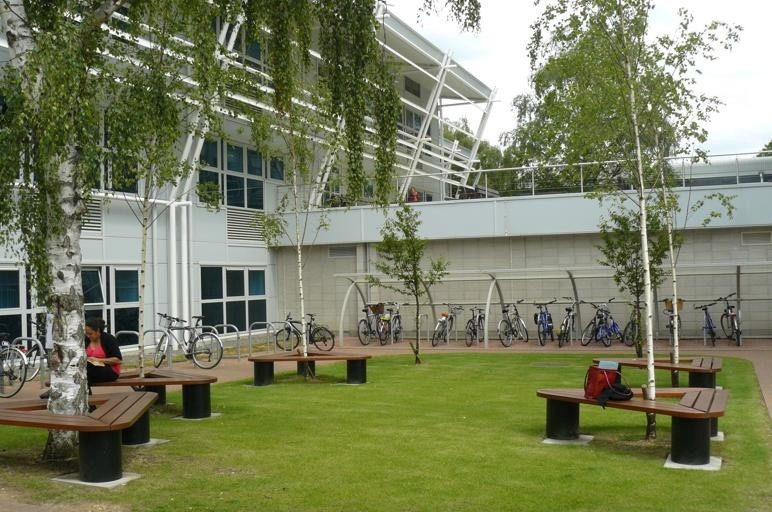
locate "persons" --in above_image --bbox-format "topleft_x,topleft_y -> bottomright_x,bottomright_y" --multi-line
85,317 -> 123,383
409,187 -> 419,201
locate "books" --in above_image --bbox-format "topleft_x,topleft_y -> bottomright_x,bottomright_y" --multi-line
87,358 -> 105,367
599,360 -> 618,369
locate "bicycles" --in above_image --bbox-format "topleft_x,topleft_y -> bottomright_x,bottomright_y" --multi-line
0,320 -> 46,398
276,312 -> 335,352
154,312 -> 223,370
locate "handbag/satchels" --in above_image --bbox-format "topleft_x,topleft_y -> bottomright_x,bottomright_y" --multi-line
584,365 -> 617,399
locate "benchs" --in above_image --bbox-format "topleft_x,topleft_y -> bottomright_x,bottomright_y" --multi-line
247,350 -> 372,389
0,363 -> 219,485
533,356 -> 731,466
